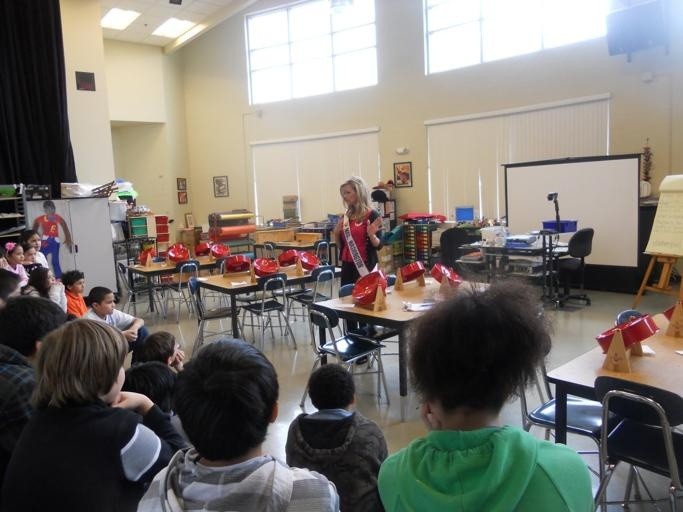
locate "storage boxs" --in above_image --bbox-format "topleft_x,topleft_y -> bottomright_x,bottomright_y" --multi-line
127,216 -> 171,242
109,201 -> 127,221
110,222 -> 126,243
60,183 -> 88,198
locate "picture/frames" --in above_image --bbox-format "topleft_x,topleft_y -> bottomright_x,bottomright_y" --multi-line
177,178 -> 186,190
393,162 -> 412,188
185,213 -> 195,228
75,71 -> 96,91
213,176 -> 229,197
178,191 -> 188,204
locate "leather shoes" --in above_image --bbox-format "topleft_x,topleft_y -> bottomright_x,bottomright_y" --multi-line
356,355 -> 371,366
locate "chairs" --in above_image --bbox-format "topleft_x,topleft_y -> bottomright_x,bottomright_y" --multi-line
517,359 -> 616,478
594,374 -> 682,510
111,226 -> 595,410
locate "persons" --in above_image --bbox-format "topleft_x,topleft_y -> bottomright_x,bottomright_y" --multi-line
32,200 -> 72,279
334,177 -> 384,365
377,275 -> 593,511
1,230 -> 388,512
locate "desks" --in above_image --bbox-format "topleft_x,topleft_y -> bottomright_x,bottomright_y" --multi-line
548,311 -> 682,448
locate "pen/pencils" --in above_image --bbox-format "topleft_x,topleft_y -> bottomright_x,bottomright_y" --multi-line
421,305 -> 434,306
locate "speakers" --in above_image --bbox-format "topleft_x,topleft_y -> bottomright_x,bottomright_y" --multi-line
606,0 -> 665,55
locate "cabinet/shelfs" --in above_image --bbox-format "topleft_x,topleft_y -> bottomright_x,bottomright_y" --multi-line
0,183 -> 27,247
24,197 -> 120,303
